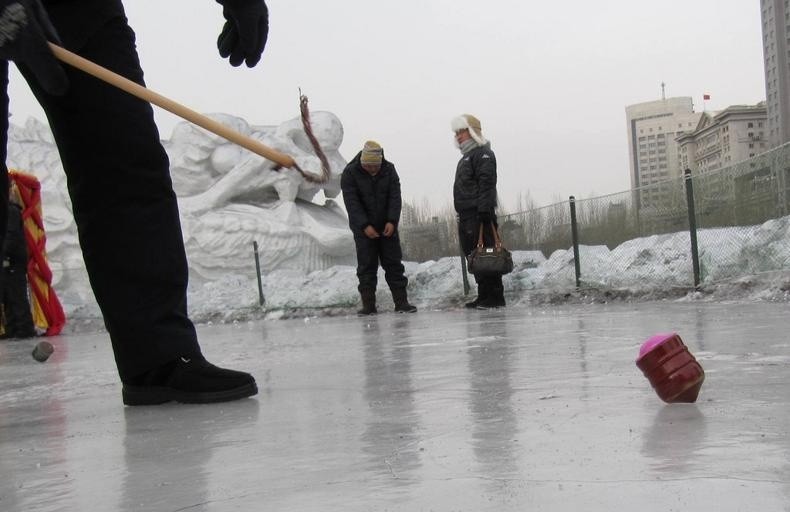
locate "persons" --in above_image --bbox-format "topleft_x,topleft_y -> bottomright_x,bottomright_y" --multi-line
0,174 -> 38,340
338,139 -> 420,317
448,113 -> 508,310
1,1 -> 275,409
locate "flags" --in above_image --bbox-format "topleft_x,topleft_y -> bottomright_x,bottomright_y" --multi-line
703,94 -> 711,100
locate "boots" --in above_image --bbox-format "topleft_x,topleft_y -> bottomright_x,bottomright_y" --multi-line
357,289 -> 377,316
389,285 -> 416,313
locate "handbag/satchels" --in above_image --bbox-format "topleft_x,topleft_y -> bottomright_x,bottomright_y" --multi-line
467,246 -> 514,276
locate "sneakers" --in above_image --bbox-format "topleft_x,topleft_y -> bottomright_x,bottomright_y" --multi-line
466,298 -> 506,308
122,347 -> 256,405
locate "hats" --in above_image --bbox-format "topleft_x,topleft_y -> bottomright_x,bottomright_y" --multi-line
452,114 -> 487,147
361,141 -> 383,165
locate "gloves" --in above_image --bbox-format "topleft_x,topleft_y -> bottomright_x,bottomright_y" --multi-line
217,1 -> 268,68
